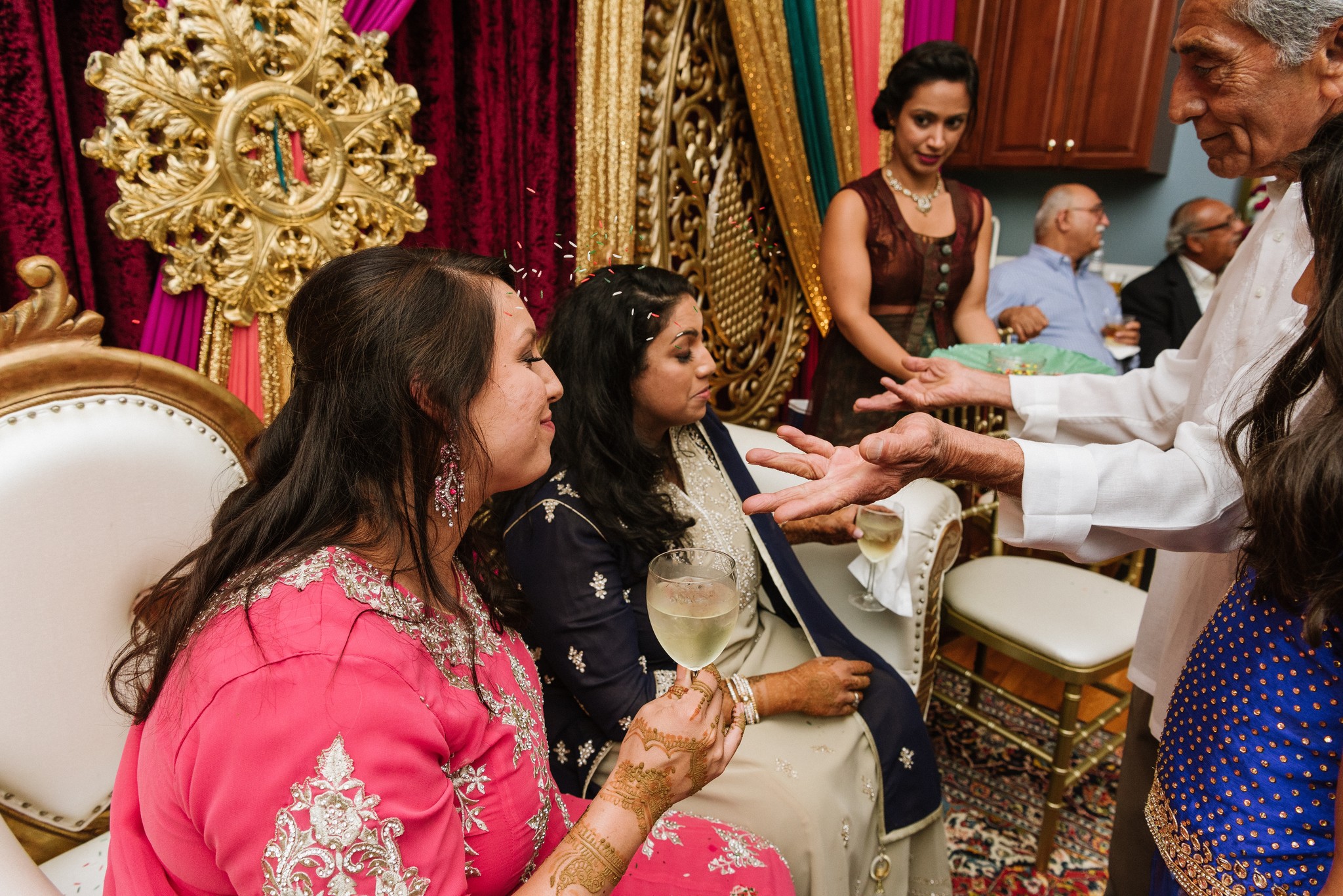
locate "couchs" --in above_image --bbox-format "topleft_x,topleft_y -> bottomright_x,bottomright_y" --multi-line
723,419 -> 963,723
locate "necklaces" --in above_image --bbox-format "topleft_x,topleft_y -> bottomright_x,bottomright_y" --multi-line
886,161 -> 942,217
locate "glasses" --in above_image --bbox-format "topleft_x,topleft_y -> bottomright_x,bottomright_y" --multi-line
1191,212 -> 1243,235
1061,205 -> 1106,217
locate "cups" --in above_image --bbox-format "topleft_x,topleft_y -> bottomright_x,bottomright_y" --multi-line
1106,313 -> 1137,345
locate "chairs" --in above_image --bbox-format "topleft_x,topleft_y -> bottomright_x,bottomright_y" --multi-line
0,255 -> 266,896
931,403 -> 1148,873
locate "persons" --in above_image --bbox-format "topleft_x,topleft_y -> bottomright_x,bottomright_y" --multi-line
453,265 -> 956,896
801,41 -> 1005,447
1118,197 -> 1248,369
985,184 -> 1142,375
739,0 -> 1342,896
1138,113 -> 1343,896
103,247 -> 797,896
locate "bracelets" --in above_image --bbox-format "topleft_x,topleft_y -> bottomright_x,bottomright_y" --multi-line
723,674 -> 761,725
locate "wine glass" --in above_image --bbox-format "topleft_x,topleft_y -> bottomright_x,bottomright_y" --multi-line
846,499 -> 904,613
646,548 -> 739,683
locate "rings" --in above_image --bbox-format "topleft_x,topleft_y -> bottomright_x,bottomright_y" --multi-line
851,691 -> 860,707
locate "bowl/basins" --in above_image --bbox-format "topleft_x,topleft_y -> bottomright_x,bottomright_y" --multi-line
988,349 -> 1048,373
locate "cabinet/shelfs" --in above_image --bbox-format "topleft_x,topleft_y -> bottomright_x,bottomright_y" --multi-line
944,0 -> 1183,178
